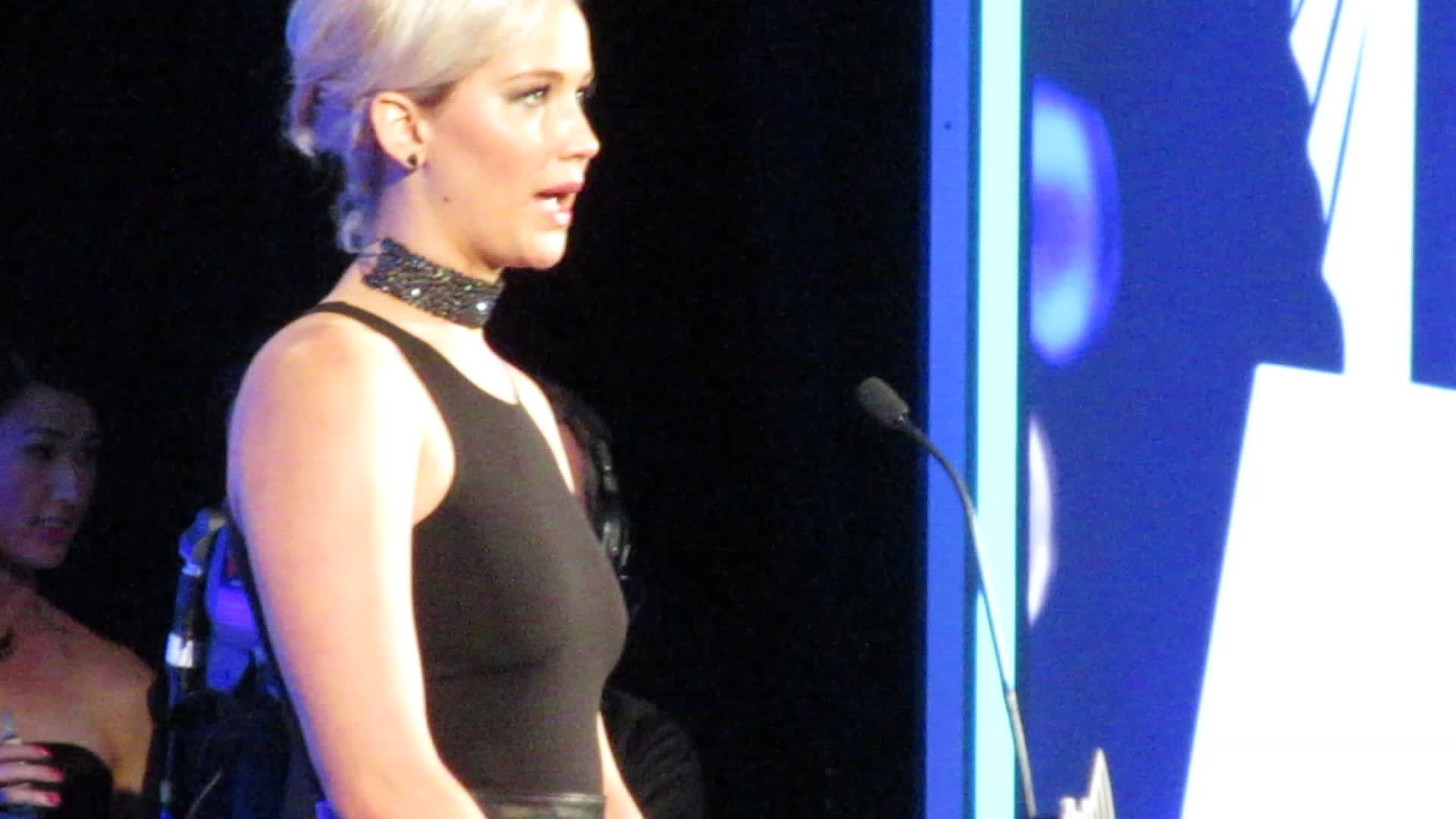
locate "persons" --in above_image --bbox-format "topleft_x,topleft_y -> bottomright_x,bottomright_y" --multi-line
163,373 -> 703,819
227,0 -> 646,819
0,340 -> 161,819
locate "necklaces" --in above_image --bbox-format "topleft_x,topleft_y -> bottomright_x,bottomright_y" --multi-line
355,233 -> 505,329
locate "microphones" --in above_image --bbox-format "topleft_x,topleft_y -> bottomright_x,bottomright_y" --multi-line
178,506 -> 226,560
859,376 -> 1037,819
